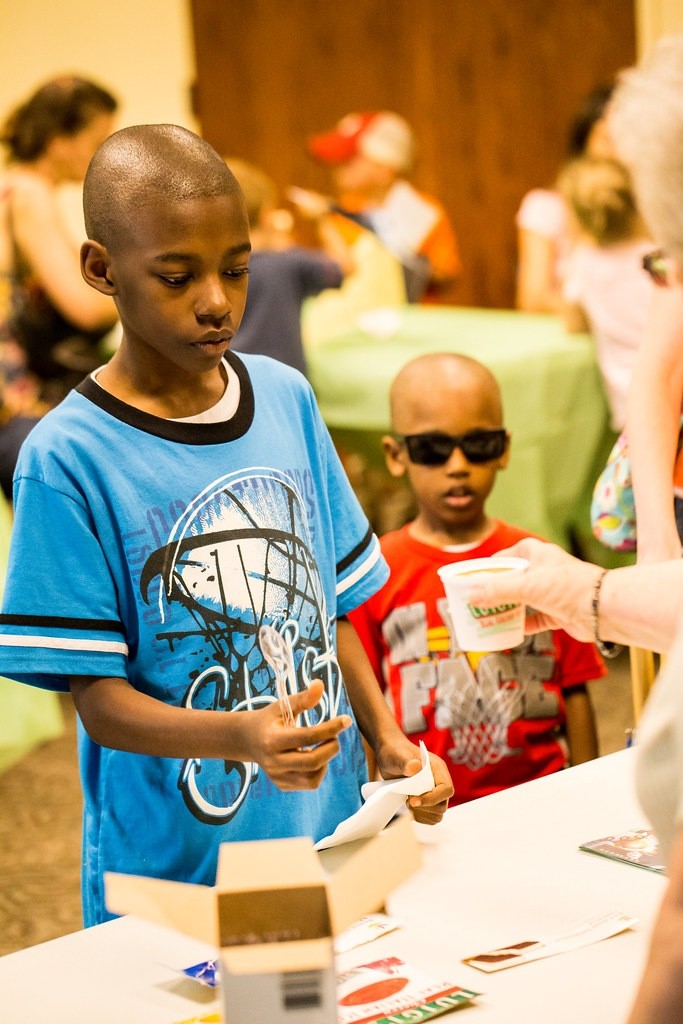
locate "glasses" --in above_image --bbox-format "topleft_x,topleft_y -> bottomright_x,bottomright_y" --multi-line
390,428 -> 509,469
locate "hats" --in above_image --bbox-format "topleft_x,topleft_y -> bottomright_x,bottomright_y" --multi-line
307,108 -> 416,174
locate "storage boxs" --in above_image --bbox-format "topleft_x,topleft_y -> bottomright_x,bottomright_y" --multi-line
103,813 -> 427,1024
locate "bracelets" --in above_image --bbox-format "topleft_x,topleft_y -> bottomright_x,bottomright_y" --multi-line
591,563 -> 623,661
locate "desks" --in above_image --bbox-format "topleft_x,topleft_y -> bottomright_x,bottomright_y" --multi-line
0,741 -> 665,1023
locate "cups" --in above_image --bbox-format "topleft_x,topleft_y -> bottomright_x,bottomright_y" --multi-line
436,555 -> 524,653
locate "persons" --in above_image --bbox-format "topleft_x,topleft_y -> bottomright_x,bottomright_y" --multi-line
1,120 -> 455,935
341,350 -> 607,819
513,73 -> 674,405
553,144 -> 674,356
301,107 -> 462,306
460,29 -> 683,1021
3,76 -> 139,517
197,143 -> 356,471
